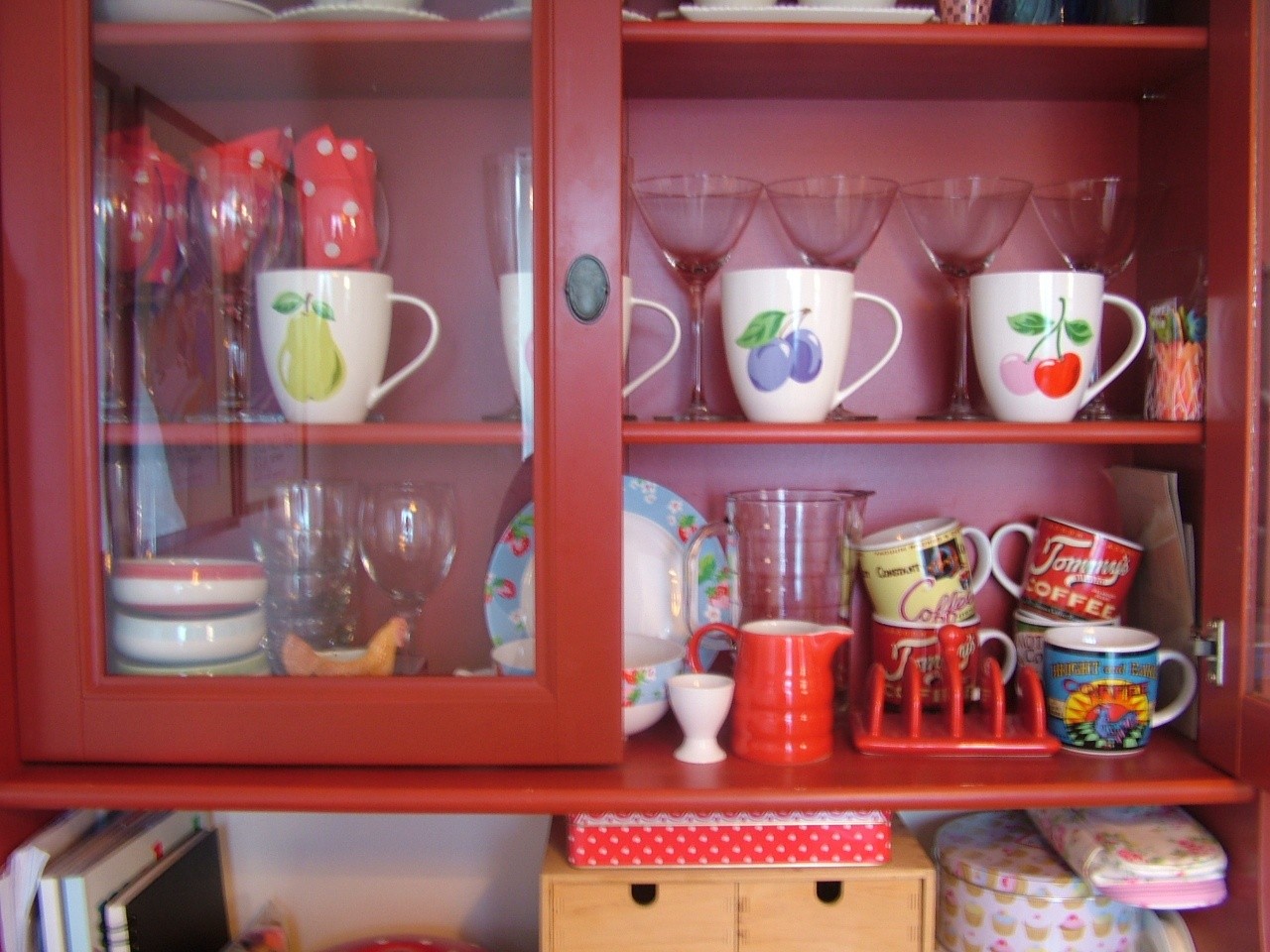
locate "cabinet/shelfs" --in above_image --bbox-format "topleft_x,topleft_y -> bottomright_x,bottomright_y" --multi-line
0,0 -> 1268,952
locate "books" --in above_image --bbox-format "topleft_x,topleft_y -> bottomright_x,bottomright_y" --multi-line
0,801 -> 241,952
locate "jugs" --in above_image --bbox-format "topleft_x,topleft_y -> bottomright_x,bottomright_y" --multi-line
688,619 -> 854,766
683,489 -> 877,718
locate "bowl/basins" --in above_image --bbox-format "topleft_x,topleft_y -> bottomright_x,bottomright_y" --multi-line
490,634 -> 686,736
105,555 -> 272,677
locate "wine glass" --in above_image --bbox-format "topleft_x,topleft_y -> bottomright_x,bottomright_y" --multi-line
87,151 -> 389,427
898,176 -> 1035,420
667,672 -> 734,765
1029,163 -> 1159,422
765,173 -> 901,423
478,144 -> 639,424
628,175 -> 764,420
354,480 -> 458,648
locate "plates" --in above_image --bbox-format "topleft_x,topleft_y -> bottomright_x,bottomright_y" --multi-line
482,474 -> 731,674
90,0 -> 936,24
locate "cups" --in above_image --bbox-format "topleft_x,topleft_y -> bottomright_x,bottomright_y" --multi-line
497,268 -> 680,410
969,270 -> 1146,423
852,511 -> 1196,757
937,0 -> 1154,25
252,268 -> 439,425
720,269 -> 904,423
254,475 -> 355,674
